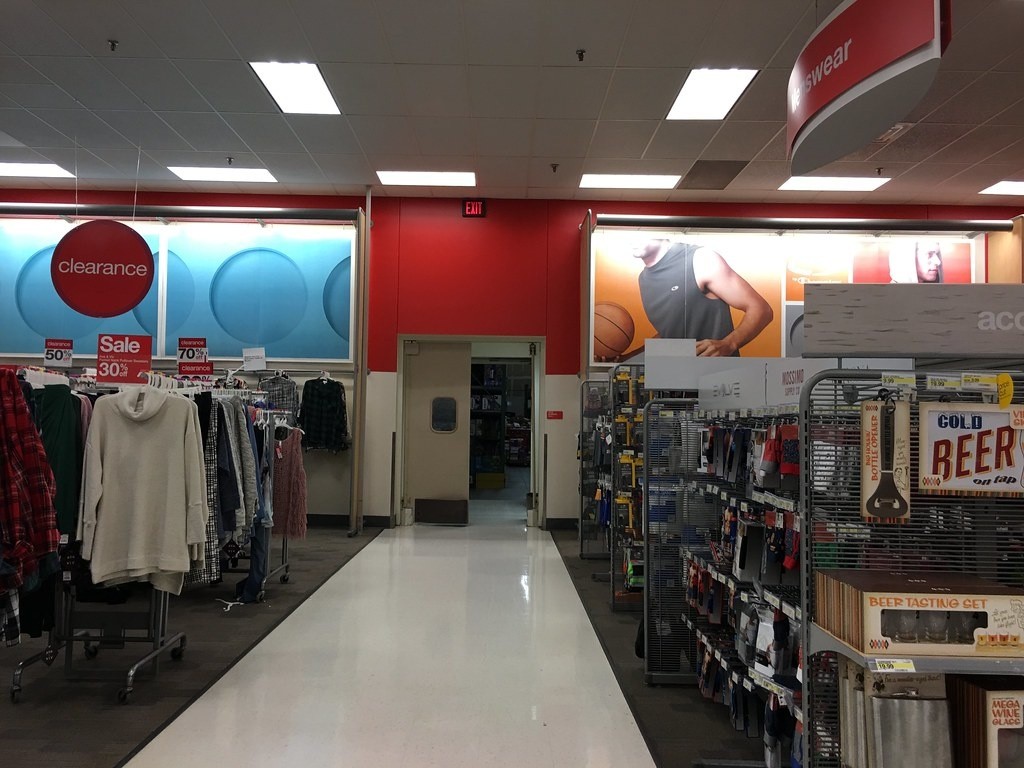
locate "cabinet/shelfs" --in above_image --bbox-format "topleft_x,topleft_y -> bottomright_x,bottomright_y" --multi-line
471,363 -> 506,473
507,428 -> 531,466
579,364 -> 866,768
802,368 -> 1024,768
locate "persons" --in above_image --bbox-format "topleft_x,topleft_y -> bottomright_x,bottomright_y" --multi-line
744,618 -> 757,644
887,241 -> 944,284
594,238 -> 774,363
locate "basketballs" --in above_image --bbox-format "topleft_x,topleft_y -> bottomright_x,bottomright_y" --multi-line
594,301 -> 635,359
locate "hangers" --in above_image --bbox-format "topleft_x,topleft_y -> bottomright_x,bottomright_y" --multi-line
16,366 -> 332,434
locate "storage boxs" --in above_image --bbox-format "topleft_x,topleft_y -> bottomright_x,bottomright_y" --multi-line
476,473 -> 505,489
813,568 -> 1024,768
